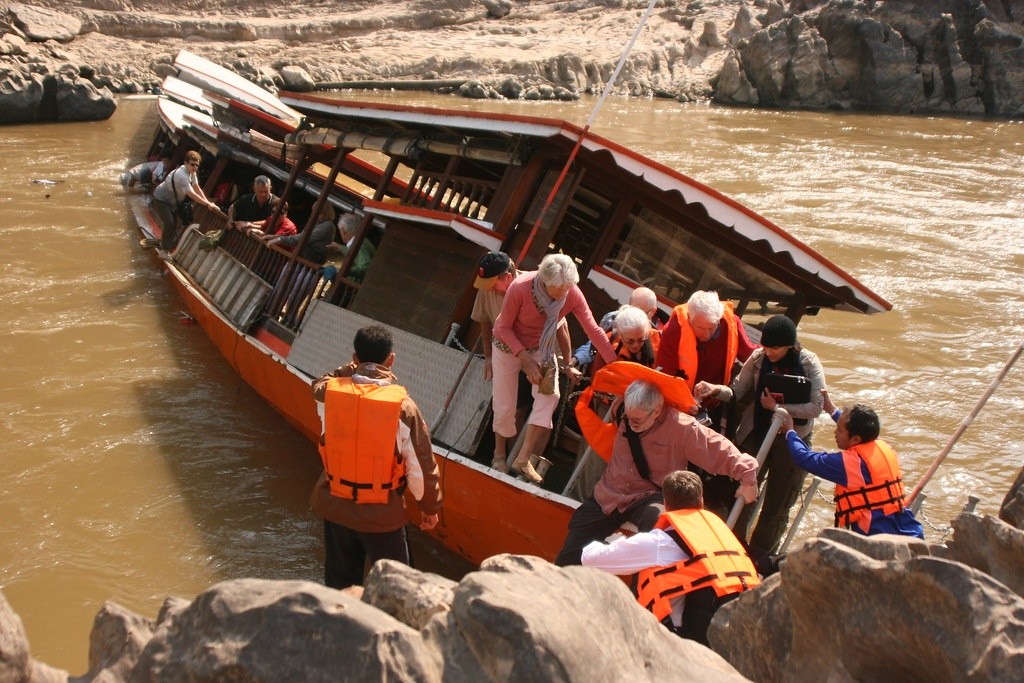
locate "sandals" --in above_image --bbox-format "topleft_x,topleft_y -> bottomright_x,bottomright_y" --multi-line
491,456 -> 544,487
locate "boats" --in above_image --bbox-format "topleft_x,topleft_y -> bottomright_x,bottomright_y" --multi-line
124,1 -> 901,599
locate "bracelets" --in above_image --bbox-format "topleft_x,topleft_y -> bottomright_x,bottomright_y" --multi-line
206,203 -> 210,208
562,361 -> 574,369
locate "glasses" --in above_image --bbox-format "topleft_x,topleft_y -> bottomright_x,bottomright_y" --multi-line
620,334 -> 647,345
190,163 -> 199,167
271,209 -> 284,215
620,409 -> 655,426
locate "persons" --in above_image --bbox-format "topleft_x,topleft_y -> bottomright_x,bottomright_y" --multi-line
152,151 -> 220,254
312,327 -> 443,590
472,251 -> 828,646
119,152 -> 179,192
780,390 -> 927,539
214,175 -> 377,324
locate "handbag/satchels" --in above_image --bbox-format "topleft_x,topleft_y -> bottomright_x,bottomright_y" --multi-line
177,199 -> 192,220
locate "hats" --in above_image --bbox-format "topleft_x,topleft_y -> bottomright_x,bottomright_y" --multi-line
473,250 -> 509,290
760,316 -> 797,348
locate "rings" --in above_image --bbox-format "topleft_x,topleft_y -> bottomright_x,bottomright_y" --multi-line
526,376 -> 530,379
